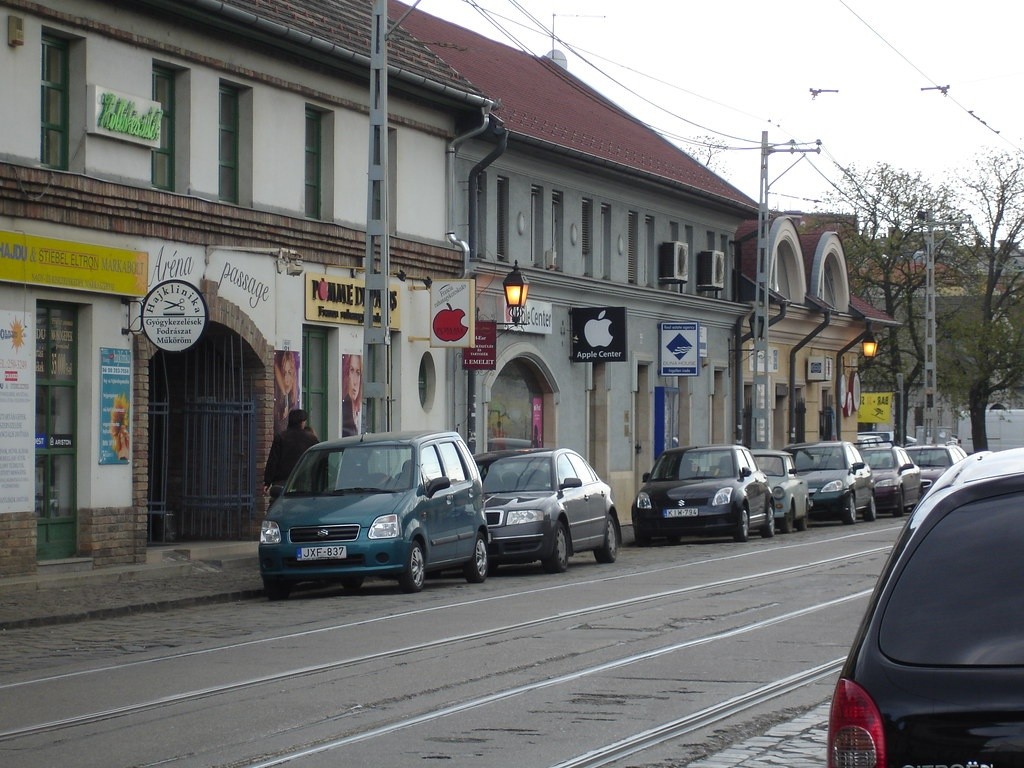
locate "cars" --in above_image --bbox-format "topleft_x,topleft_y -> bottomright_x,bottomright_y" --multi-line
258,429 -> 490,599
631,443 -> 775,548
826,444 -> 1024,768
780,439 -> 877,526
900,439 -> 967,500
851,441 -> 922,518
749,448 -> 811,534
480,448 -> 623,577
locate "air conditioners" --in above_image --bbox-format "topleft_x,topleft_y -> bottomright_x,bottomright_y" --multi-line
658,241 -> 690,284
695,250 -> 726,290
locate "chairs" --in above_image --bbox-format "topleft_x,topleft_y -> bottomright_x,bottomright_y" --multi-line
717,455 -> 733,476
394,459 -> 414,489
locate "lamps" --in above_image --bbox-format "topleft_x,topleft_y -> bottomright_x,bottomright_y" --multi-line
842,333 -> 879,368
474,260 -> 530,326
728,311 -> 763,352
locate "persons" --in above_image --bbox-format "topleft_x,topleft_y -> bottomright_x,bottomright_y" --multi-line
260,409 -> 320,500
341,353 -> 362,437
274,351 -> 300,428
